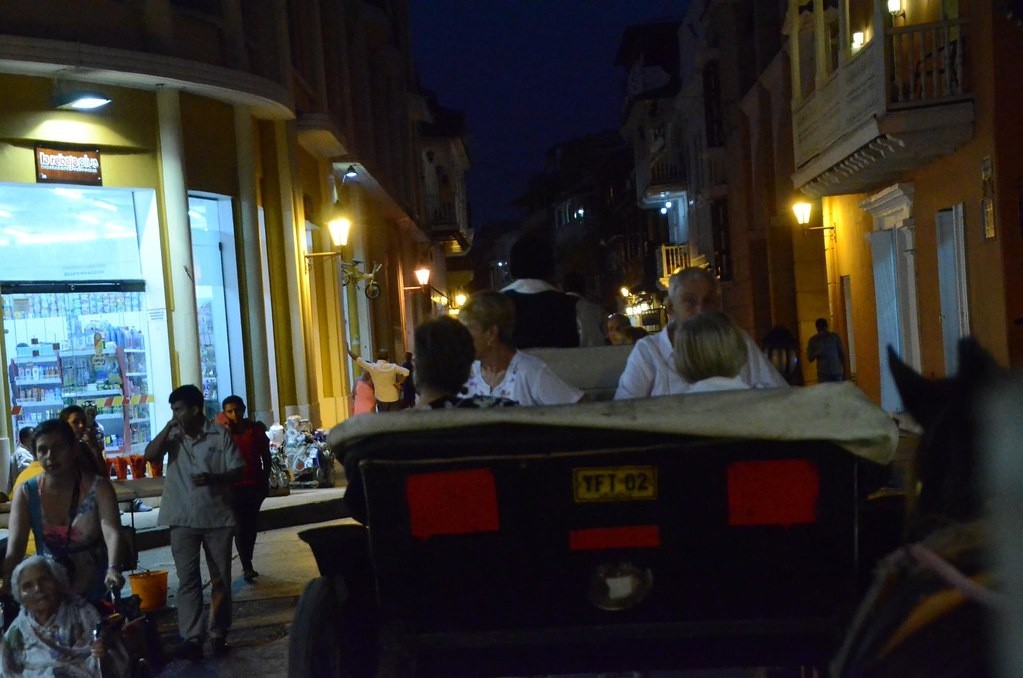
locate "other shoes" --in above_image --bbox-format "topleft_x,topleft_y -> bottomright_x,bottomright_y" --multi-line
201,637 -> 224,658
174,641 -> 200,662
245,571 -> 258,583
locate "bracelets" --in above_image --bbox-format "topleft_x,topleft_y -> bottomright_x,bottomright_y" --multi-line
108,563 -> 122,569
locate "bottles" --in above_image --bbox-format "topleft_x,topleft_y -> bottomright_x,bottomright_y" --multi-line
130,424 -> 151,444
19,365 -> 58,379
130,405 -> 148,419
124,352 -> 146,372
84,321 -> 145,350
63,353 -> 122,391
64,397 -> 123,414
106,434 -> 124,447
23,409 -> 62,422
203,380 -> 217,400
20,387 -> 60,402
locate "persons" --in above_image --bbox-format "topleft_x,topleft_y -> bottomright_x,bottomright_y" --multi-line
806,318 -> 848,383
675,311 -> 755,391
503,241 -> 607,346
351,361 -> 377,415
605,313 -> 649,344
611,267 -> 789,401
215,395 -> 271,579
347,343 -> 410,413
0,420 -> 125,614
396,316 -> 520,413
402,352 -> 415,409
146,385 -> 247,658
461,292 -> 585,408
6,426 -> 48,558
0,556 -> 129,678
61,400 -> 108,478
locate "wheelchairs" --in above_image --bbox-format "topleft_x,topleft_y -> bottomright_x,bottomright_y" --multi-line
0,581 -> 153,678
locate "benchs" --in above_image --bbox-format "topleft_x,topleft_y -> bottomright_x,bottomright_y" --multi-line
326,343 -> 899,627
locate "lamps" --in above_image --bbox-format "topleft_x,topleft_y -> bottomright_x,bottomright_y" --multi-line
402,263 -> 430,293
794,196 -> 836,242
345,164 -> 358,177
434,292 -> 467,316
661,204 -> 667,214
666,195 -> 671,208
304,218 -> 351,275
49,78 -> 113,112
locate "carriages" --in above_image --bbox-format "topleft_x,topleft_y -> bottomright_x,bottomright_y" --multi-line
283,336 -> 1023,678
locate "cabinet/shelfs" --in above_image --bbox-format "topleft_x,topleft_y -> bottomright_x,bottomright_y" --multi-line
10,344 -> 218,456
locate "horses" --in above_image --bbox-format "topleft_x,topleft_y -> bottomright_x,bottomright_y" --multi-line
827,332 -> 1023,678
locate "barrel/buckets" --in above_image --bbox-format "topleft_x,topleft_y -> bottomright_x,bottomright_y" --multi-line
128,570 -> 168,609
105,455 -> 163,479
